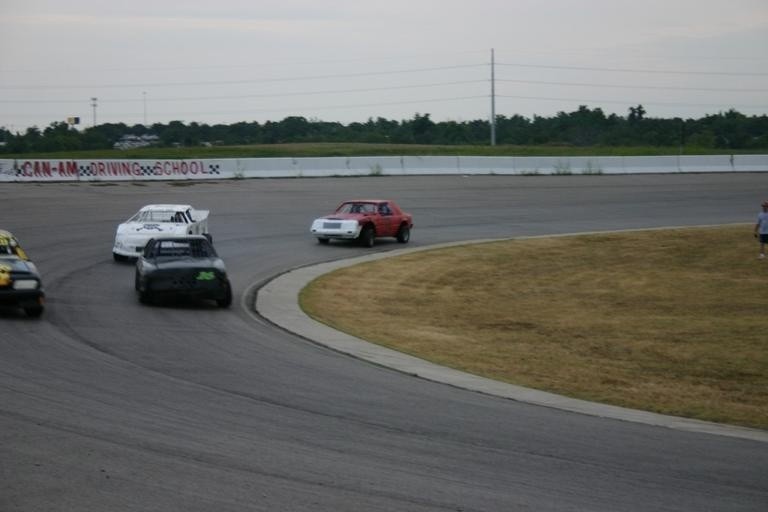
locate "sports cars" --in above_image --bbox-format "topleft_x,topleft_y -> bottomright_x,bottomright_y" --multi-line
109,202 -> 212,263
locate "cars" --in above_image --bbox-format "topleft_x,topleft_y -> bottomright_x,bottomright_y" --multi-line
307,197 -> 414,250
0,226 -> 47,324
134,234 -> 235,313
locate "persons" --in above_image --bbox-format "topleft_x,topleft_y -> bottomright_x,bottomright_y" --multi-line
752,201 -> 768,261
